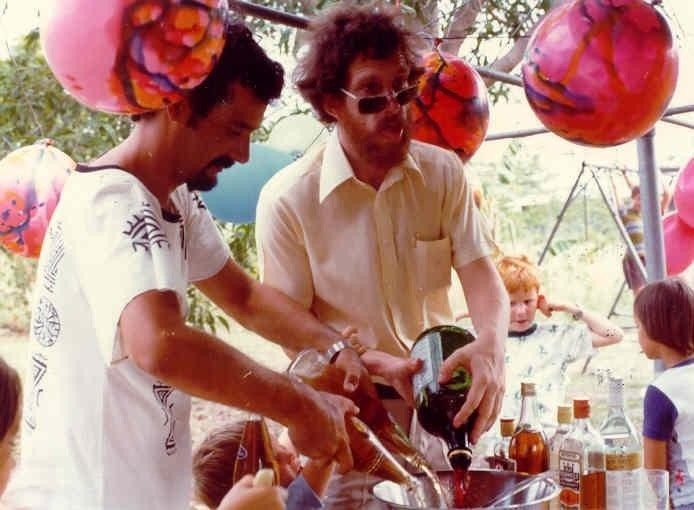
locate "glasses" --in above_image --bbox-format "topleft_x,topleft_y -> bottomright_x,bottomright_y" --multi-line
338,79 -> 419,115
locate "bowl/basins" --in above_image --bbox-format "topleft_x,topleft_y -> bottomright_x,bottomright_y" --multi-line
368,468 -> 562,510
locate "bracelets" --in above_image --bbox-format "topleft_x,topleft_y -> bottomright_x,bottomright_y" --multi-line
324,340 -> 353,366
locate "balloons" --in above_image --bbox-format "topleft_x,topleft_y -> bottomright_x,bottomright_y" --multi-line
520,0 -> 680,148
401,38 -> 489,166
198,112 -> 329,225
661,158 -> 694,278
33,1 -> 228,118
0,138 -> 77,259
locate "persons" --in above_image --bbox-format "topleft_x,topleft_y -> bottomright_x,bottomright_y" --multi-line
191,326 -> 366,509
629,279 -> 694,510
611,180 -> 670,292
451,257 -> 623,423
252,1 -> 511,473
0,356 -> 289,509
18,9 -> 377,509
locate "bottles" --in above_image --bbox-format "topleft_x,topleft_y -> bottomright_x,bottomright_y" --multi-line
283,347 -> 431,497
232,410 -> 282,487
493,377 -> 640,510
408,325 -> 477,471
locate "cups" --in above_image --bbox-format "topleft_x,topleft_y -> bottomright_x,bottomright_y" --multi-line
641,469 -> 670,510
